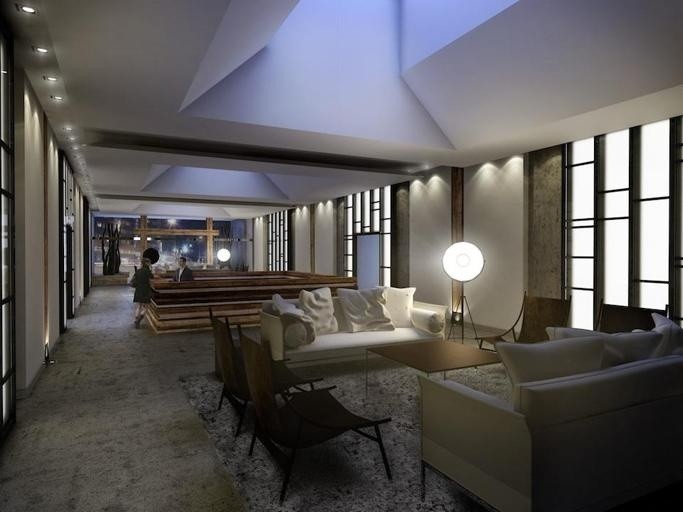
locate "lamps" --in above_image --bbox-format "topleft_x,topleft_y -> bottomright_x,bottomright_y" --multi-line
443,242 -> 485,348
217,249 -> 233,271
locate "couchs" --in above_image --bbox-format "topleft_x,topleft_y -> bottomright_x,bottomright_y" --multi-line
416,327 -> 683,512
260,296 -> 449,369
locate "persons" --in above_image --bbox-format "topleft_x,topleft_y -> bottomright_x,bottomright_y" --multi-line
131,258 -> 159,328
173,257 -> 193,281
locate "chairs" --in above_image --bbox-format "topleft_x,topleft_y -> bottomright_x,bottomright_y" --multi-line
207,306 -> 393,506
593,298 -> 669,334
475,290 -> 571,368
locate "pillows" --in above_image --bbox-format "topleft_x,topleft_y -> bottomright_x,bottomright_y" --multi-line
263,285 -> 445,348
496,313 -> 683,389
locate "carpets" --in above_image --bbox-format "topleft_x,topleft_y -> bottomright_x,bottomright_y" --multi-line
180,363 -> 513,512
